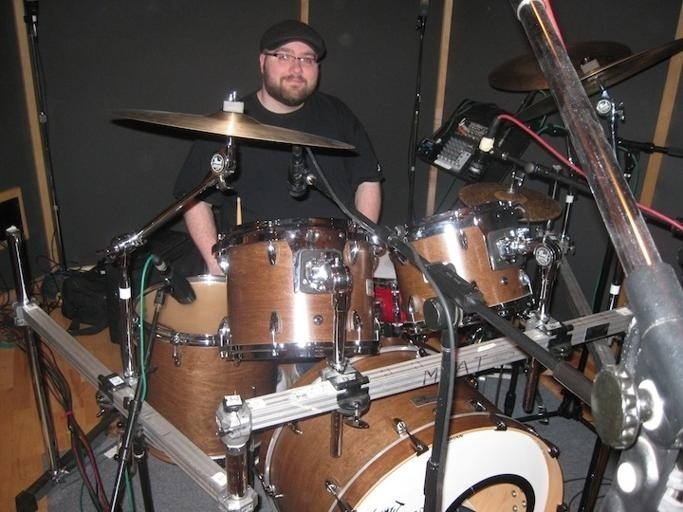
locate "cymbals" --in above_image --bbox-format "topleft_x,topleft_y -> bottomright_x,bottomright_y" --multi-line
99,107 -> 356,151
459,182 -> 563,223
488,40 -> 632,93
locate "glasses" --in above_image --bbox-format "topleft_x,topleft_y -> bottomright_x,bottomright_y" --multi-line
262,52 -> 316,64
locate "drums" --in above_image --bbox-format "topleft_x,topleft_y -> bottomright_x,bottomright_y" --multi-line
372,198 -> 539,337
132,273 -> 277,465
254,344 -> 565,512
227,216 -> 378,361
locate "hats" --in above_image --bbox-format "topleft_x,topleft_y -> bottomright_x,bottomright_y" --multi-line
259,19 -> 328,62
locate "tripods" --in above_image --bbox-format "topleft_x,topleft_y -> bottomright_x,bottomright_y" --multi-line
0,9 -> 683,512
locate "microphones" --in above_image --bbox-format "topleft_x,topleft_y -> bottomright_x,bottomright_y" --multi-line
287,144 -> 308,198
152,254 -> 196,305
464,118 -> 500,180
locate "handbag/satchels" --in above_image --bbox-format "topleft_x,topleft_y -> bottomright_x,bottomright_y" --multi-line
61,229 -> 202,337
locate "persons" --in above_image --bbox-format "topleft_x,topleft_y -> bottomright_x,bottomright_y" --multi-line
173,19 -> 383,278
